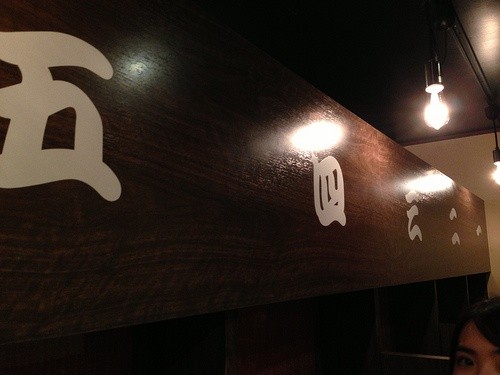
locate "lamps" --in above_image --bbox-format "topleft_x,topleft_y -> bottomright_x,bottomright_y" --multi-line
485,104 -> 500,185
423,11 -> 450,131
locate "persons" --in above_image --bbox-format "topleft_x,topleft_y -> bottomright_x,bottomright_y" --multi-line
445,295 -> 500,375
